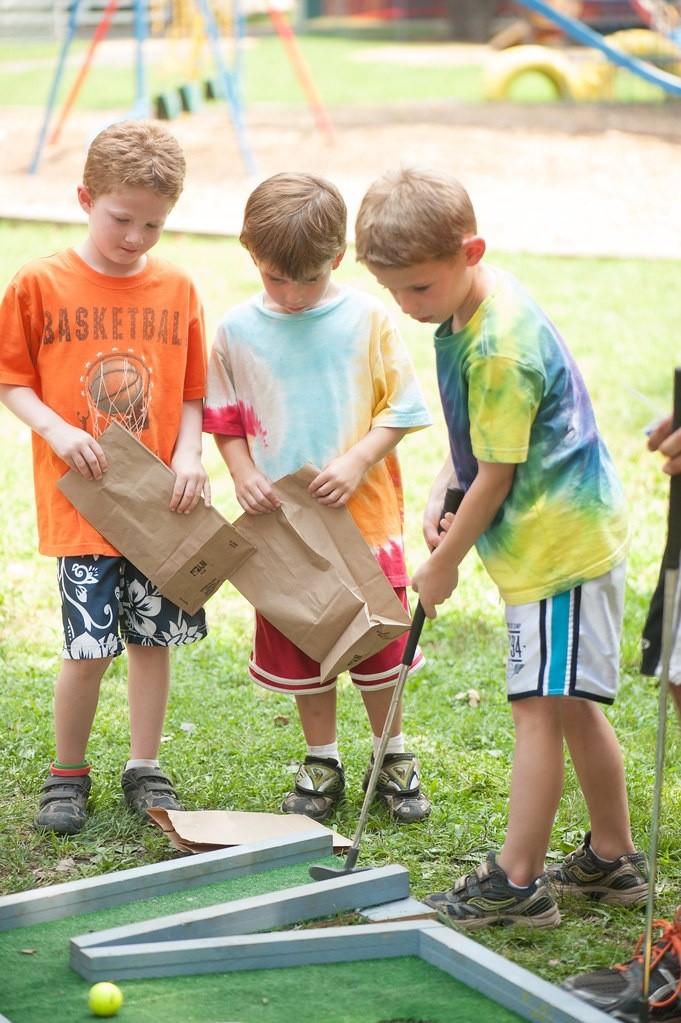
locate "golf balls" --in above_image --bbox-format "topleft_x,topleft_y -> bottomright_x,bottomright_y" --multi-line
87,980 -> 125,1017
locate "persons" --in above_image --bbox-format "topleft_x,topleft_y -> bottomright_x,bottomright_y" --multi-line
201,172 -> 435,823
0,120 -> 212,835
355,166 -> 660,929
557,413 -> 681,1023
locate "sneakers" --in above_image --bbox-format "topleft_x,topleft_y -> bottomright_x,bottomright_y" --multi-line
280,756 -> 346,822
545,832 -> 658,908
422,850 -> 562,932
34,773 -> 93,834
121,762 -> 180,822
361,750 -> 431,823
560,904 -> 680,1022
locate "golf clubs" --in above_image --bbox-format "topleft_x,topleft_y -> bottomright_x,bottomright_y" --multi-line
306,485 -> 467,883
611,362 -> 681,1023
